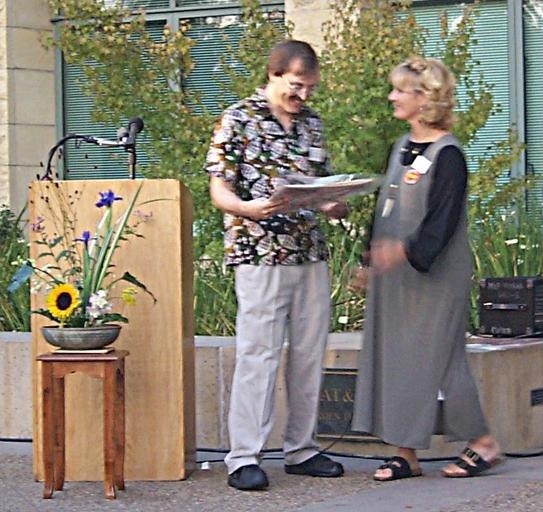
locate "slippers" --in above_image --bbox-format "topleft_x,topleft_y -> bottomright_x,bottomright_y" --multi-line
441,445 -> 505,479
373,453 -> 424,482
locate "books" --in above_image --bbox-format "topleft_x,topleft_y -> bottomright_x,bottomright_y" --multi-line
269,171 -> 374,209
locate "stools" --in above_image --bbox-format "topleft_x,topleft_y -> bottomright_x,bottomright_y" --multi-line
33,349 -> 132,500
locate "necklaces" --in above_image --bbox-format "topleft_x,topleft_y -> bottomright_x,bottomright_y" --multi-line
381,130 -> 439,219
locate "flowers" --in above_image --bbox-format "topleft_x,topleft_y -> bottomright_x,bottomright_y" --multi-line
10,148 -> 174,330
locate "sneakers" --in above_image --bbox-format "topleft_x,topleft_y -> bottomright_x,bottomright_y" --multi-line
227,464 -> 271,491
284,452 -> 345,478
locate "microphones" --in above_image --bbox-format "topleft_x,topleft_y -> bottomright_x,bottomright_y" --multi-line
118,127 -> 137,179
126,117 -> 145,179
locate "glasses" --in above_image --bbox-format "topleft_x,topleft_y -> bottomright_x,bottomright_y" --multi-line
282,75 -> 318,92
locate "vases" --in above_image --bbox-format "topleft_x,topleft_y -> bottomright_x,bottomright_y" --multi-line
40,324 -> 123,350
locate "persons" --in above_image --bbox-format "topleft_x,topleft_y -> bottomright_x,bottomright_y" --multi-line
351,56 -> 505,481
206,40 -> 355,489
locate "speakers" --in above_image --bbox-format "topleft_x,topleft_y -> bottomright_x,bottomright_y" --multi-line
478,277 -> 542,339
